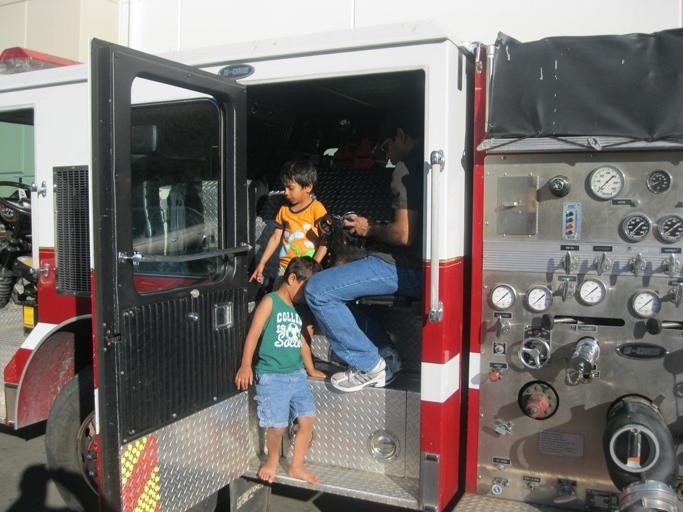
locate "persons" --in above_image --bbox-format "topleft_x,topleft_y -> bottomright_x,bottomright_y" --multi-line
303,119 -> 422,393
247,158 -> 334,349
234,256 -> 329,485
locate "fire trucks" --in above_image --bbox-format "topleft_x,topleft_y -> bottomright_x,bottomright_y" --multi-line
0,29 -> 683,512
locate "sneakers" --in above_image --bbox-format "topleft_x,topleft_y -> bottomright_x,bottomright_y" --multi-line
330,346 -> 403,392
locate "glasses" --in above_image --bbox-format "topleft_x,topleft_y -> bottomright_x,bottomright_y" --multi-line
381,137 -> 391,151
